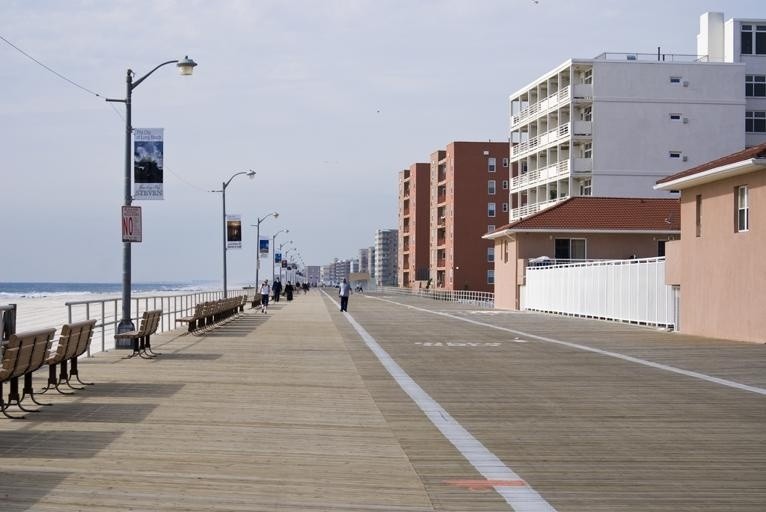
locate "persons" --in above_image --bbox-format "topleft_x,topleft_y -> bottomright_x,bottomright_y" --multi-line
338,277 -> 353,312
272,277 -> 282,302
295,281 -> 310,295
284,280 -> 294,301
259,279 -> 270,313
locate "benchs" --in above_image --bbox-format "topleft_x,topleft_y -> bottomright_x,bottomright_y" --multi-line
0,325 -> 55,421
172,288 -> 286,337
31,318 -> 100,397
109,307 -> 167,360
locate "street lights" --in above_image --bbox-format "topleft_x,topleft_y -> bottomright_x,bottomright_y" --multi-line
114,54 -> 199,349
279,240 -> 305,287
256,211 -> 280,293
272,229 -> 289,287
221,170 -> 256,300
